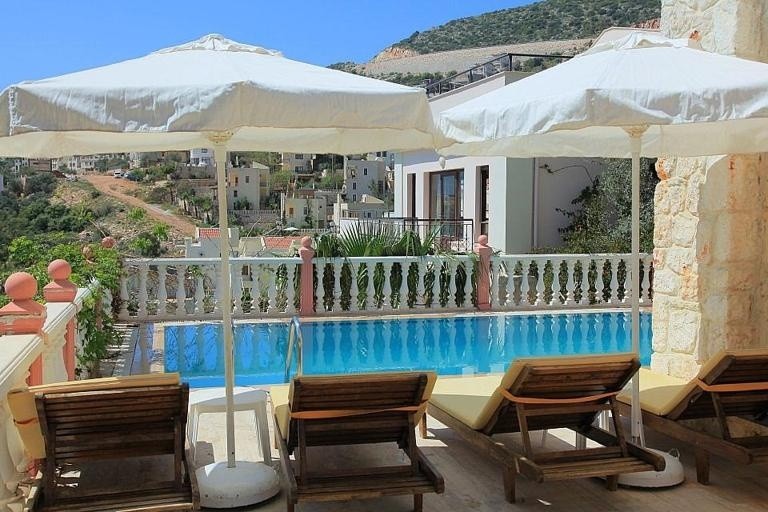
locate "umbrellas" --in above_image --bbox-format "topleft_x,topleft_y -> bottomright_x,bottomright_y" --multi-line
434,24 -> 768,448
0,33 -> 432,468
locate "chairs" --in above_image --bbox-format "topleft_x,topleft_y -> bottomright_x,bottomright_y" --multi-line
419,353 -> 666,503
7,372 -> 201,512
615,346 -> 768,486
275,370 -> 444,512
